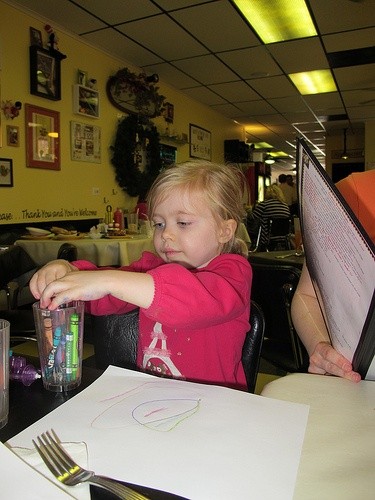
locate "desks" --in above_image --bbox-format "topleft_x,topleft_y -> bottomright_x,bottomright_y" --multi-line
0,353 -> 375,500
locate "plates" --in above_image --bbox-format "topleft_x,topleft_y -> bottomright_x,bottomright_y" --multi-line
21,235 -> 53,239
106,236 -> 131,239
53,234 -> 81,239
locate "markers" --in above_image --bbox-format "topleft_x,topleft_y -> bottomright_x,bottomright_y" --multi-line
43,314 -> 80,383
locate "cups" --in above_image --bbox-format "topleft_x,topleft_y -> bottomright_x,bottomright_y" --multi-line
107,228 -> 122,236
0,317 -> 9,428
32,298 -> 85,392
293,218 -> 304,257
126,213 -> 138,234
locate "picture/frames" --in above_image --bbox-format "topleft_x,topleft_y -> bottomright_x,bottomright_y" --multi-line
70,120 -> 102,163
0,103 -> 61,187
189,123 -> 212,161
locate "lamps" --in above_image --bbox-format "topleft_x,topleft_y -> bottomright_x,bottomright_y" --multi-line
342,129 -> 349,159
265,151 -> 276,164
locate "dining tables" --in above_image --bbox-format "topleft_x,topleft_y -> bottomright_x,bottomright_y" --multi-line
14,238 -> 155,266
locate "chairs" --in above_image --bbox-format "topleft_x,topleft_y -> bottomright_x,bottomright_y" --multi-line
267,220 -> 291,250
0,246 -> 310,394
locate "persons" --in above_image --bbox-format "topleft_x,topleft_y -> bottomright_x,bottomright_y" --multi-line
291,168 -> 375,381
278,174 -> 297,207
30,162 -> 253,391
245,186 -> 290,252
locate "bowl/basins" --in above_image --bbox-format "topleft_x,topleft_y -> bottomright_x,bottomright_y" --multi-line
25,226 -> 51,236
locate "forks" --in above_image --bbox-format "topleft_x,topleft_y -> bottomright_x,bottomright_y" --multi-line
31,432 -> 149,499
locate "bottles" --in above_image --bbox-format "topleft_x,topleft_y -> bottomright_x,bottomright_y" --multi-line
114,208 -> 123,230
